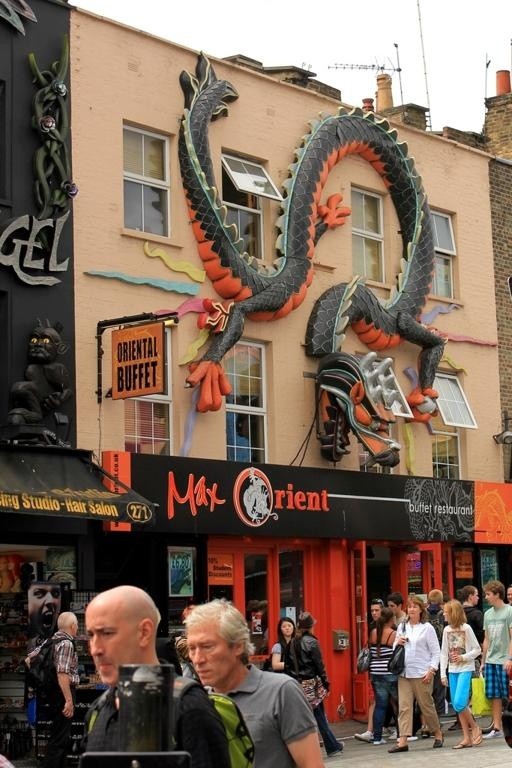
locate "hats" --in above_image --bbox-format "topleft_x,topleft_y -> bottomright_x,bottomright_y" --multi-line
295,611 -> 317,629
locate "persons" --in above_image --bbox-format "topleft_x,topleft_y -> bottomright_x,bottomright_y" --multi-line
272,618 -> 295,673
186,600 -> 325,768
387,593 -> 406,624
25,612 -> 79,768
27,582 -> 61,636
81,584 -> 227,768
506,585 -> 512,605
479,583 -> 512,741
457,585 -> 485,651
368,607 -> 418,745
420,590 -> 448,738
388,597 -> 444,753
284,612 -> 345,758
440,600 -> 482,749
354,599 -> 398,742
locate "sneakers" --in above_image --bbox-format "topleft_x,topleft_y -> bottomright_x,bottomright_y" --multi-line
388,731 -> 398,740
354,729 -> 375,743
481,728 -> 504,740
373,737 -> 387,745
328,741 -> 345,757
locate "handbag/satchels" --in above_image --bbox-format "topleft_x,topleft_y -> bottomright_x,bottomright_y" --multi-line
262,641 -> 284,673
356,643 -> 370,674
470,671 -> 494,718
387,622 -> 406,676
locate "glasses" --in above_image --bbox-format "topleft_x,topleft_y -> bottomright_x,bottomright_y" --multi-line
371,598 -> 385,606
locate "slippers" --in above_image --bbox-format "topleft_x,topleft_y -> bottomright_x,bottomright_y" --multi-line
472,729 -> 483,746
451,738 -> 471,750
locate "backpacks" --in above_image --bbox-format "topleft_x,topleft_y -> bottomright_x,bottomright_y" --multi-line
30,637 -> 77,699
202,686 -> 256,768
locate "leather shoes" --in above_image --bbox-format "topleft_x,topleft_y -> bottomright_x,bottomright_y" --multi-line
389,744 -> 409,753
432,736 -> 445,751
